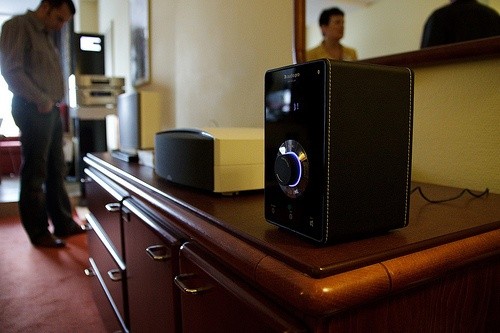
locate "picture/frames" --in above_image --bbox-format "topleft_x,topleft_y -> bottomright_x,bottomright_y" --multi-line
128,0 -> 152,88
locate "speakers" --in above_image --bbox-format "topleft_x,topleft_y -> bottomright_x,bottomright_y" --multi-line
264,58 -> 414,244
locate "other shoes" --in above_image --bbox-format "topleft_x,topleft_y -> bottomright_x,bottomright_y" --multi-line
31,233 -> 66,249
53,225 -> 86,238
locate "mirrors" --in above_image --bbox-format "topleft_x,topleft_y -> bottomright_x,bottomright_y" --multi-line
291,0 -> 500,70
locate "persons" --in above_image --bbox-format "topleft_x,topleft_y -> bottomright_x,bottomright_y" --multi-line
421,0 -> 500,50
306,7 -> 357,63
0,0 -> 87,248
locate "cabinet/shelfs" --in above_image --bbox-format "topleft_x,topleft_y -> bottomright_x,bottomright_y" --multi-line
80,151 -> 500,333
